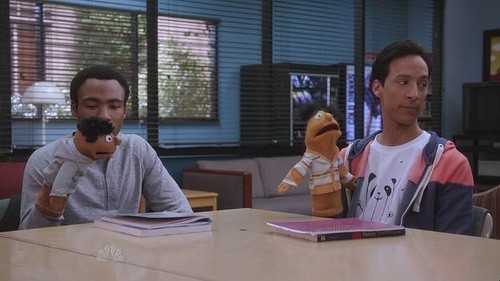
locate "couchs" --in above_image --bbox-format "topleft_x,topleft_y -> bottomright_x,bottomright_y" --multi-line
179,155 -> 314,216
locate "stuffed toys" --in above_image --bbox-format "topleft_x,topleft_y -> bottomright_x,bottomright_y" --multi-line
35,116 -> 122,217
277,99 -> 356,217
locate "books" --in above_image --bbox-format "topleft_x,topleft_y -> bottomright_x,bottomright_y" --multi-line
267,222 -> 406,243
94,221 -> 212,238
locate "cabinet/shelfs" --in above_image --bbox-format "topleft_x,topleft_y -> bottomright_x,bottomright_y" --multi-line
238,62 -> 348,151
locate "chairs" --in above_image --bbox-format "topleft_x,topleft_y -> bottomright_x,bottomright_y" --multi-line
0,194 -> 21,232
471,205 -> 493,238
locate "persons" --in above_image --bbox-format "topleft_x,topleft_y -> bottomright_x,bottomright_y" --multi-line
340,40 -> 474,236
19,66 -> 193,229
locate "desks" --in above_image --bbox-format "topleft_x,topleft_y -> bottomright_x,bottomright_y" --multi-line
0,206 -> 500,281
139,188 -> 219,215
450,133 -> 500,182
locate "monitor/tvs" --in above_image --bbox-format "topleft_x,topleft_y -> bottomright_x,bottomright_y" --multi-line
462,82 -> 500,133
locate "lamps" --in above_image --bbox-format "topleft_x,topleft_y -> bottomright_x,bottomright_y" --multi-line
19,81 -> 66,149
